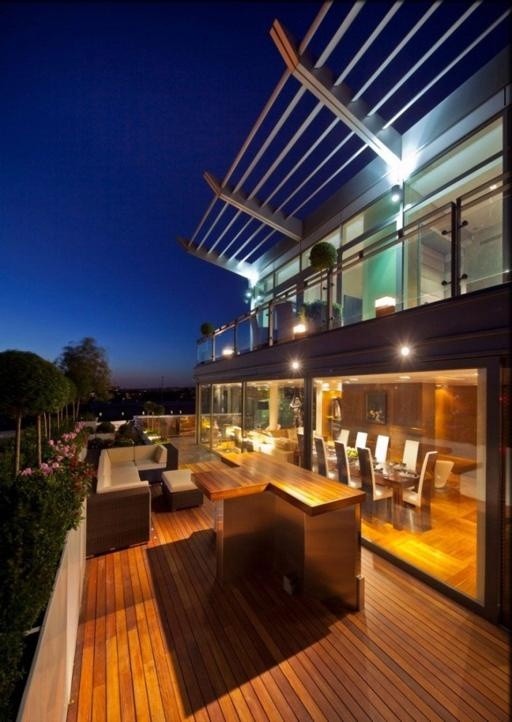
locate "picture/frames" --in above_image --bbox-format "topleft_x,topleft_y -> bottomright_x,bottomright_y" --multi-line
364,390 -> 389,427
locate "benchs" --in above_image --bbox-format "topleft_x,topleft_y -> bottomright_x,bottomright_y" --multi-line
434,453 -> 485,502
162,468 -> 204,511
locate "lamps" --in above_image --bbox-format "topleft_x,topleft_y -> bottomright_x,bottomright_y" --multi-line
390,184 -> 402,202
375,296 -> 395,319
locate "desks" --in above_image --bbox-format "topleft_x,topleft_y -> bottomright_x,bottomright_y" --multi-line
190,453 -> 367,612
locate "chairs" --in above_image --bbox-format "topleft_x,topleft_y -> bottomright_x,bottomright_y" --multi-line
297,429 -> 439,531
232,425 -> 289,453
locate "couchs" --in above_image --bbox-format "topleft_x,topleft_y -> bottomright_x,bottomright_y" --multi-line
87,442 -> 178,556
